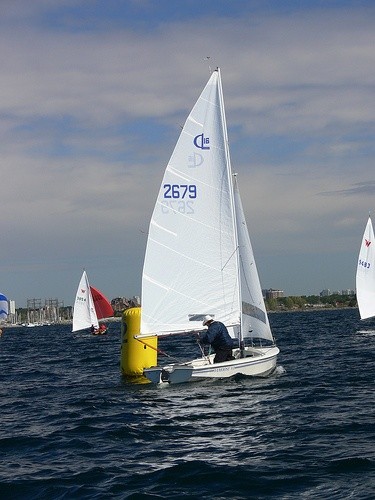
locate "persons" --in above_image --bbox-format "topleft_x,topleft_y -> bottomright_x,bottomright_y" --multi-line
91,324 -> 95,332
200,315 -> 236,363
100,323 -> 106,330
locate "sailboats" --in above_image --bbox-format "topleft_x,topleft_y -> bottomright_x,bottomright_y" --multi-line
356,212 -> 375,338
71,269 -> 114,336
135,66 -> 282,390
0,293 -> 8,338
20,306 -> 61,328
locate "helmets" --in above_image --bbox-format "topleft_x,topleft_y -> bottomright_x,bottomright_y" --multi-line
203,315 -> 213,326
